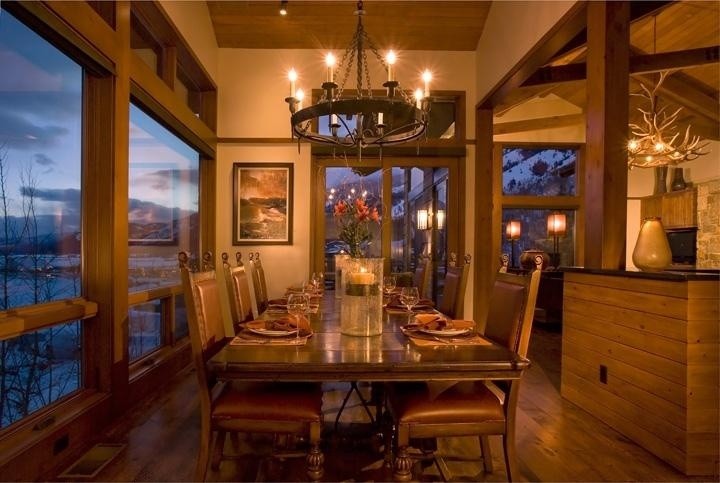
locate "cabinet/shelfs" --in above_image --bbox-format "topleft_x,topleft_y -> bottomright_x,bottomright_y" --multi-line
639,190 -> 698,231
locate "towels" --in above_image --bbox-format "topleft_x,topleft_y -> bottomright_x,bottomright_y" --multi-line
404,315 -> 477,329
268,298 -> 287,306
242,312 -> 313,337
386,294 -> 432,307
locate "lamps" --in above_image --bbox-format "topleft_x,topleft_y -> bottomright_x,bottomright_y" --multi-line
417,208 -> 428,230
286,1 -> 429,163
546,213 -> 567,268
436,209 -> 447,229
626,14 -> 711,171
505,218 -> 521,268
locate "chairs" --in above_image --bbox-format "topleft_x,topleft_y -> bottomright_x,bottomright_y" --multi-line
222,251 -> 255,336
409,255 -> 432,299
250,251 -> 268,320
383,254 -> 546,482
435,253 -> 471,321
178,252 -> 326,482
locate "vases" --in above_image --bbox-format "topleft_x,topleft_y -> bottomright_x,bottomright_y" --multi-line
341,256 -> 383,337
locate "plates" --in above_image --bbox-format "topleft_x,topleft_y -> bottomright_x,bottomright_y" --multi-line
249,322 -> 299,337
420,322 -> 470,336
400,287 -> 419,327
397,304 -> 430,309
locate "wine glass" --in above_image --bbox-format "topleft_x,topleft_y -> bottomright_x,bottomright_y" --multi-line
303,281 -> 316,315
312,272 -> 324,300
287,293 -> 308,344
382,276 -> 396,306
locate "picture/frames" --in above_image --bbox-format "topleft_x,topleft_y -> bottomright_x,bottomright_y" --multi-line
232,162 -> 294,246
60,161 -> 186,245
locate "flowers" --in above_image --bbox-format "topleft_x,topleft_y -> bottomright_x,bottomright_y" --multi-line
332,196 -> 383,256
